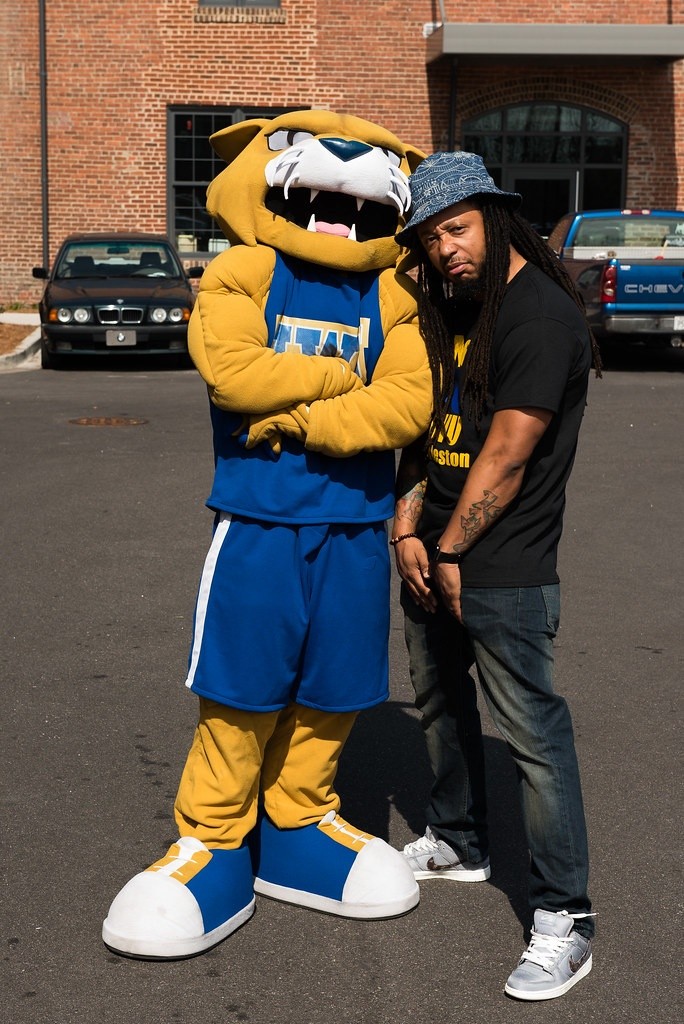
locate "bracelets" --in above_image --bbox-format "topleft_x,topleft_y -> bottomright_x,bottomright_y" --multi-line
389,533 -> 416,546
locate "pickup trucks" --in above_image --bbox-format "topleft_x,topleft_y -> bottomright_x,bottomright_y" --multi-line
547,210 -> 684,370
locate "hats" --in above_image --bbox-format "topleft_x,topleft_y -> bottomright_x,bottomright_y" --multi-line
394,151 -> 522,247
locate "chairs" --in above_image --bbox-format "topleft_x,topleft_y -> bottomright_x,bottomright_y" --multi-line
72,256 -> 94,276
139,251 -> 160,268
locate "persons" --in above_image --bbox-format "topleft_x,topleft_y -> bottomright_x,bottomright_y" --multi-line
390,152 -> 604,999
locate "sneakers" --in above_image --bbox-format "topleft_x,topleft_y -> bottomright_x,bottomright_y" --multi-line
399,825 -> 490,882
505,908 -> 599,1000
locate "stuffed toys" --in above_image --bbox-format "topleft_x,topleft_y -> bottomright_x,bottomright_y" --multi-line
103,112 -> 443,957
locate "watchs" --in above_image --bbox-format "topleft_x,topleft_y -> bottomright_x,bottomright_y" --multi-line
433,544 -> 460,563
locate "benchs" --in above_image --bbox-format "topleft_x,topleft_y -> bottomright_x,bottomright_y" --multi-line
95,263 -> 139,275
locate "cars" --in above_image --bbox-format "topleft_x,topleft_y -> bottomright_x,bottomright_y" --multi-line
32,231 -> 206,372
